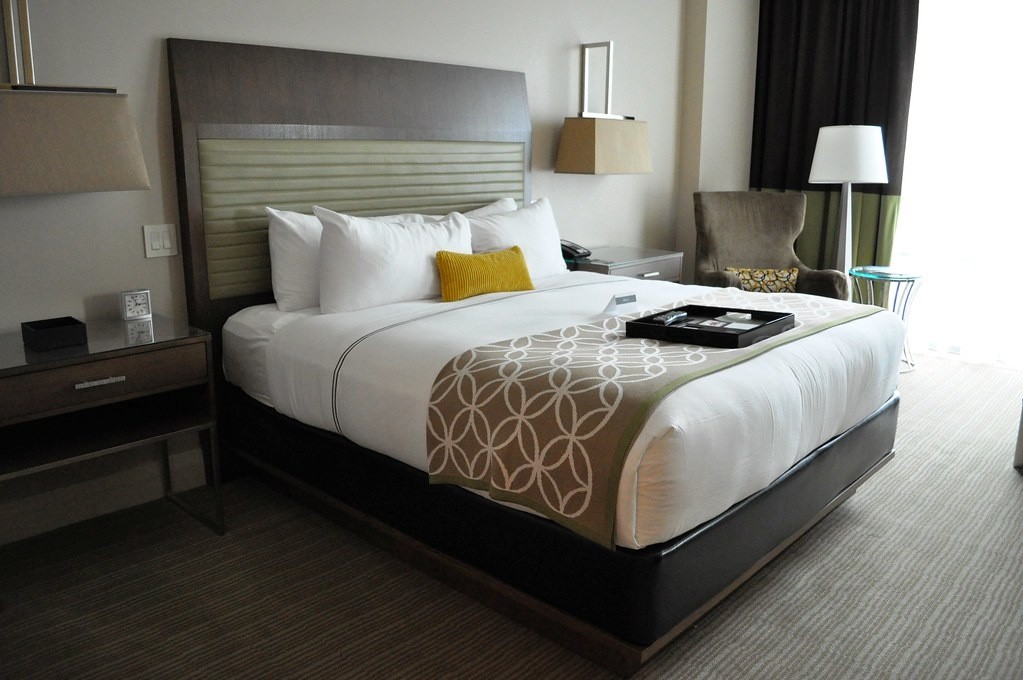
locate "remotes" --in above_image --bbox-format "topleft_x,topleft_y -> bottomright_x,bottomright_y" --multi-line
654,309 -> 688,326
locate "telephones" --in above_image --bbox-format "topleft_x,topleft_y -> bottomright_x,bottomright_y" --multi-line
560,238 -> 592,260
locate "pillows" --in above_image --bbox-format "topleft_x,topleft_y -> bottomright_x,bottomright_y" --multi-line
724,266 -> 799,295
264,195 -> 571,314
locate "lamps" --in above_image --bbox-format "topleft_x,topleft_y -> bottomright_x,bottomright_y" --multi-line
554,41 -> 652,174
0,0 -> 154,198
808,124 -> 889,303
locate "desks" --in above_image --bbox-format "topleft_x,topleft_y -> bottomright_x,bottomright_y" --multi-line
0,311 -> 228,549
849,264 -> 924,373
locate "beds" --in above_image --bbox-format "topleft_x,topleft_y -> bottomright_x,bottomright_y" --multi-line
166,36 -> 908,680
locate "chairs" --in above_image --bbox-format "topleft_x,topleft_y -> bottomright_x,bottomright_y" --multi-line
693,190 -> 849,303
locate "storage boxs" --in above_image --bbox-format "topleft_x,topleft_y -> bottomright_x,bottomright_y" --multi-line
21,316 -> 87,353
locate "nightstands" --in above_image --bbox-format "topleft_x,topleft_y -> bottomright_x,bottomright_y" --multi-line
575,246 -> 684,286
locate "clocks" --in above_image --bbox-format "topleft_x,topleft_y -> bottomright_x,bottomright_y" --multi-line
127,319 -> 154,345
120,289 -> 153,320
